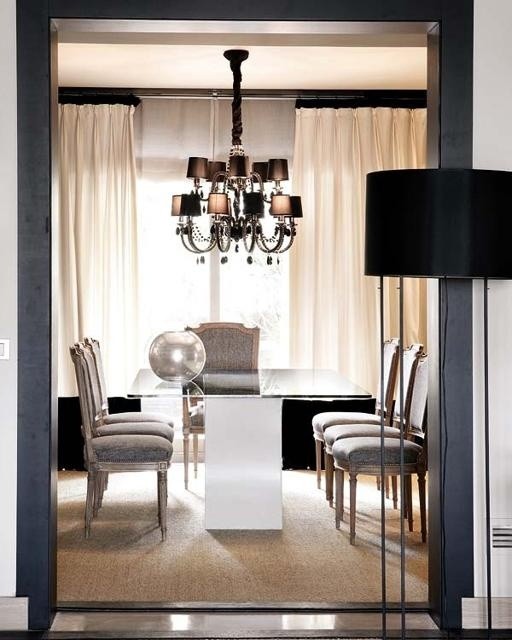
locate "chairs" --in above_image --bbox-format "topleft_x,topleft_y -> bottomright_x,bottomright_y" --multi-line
184,322 -> 260,489
333,356 -> 429,542
85,336 -> 175,427
314,336 -> 400,501
324,344 -> 424,522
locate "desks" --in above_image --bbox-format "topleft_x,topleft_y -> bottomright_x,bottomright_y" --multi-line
126,370 -> 373,530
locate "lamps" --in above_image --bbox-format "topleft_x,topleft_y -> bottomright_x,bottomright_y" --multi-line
170,148 -> 304,266
